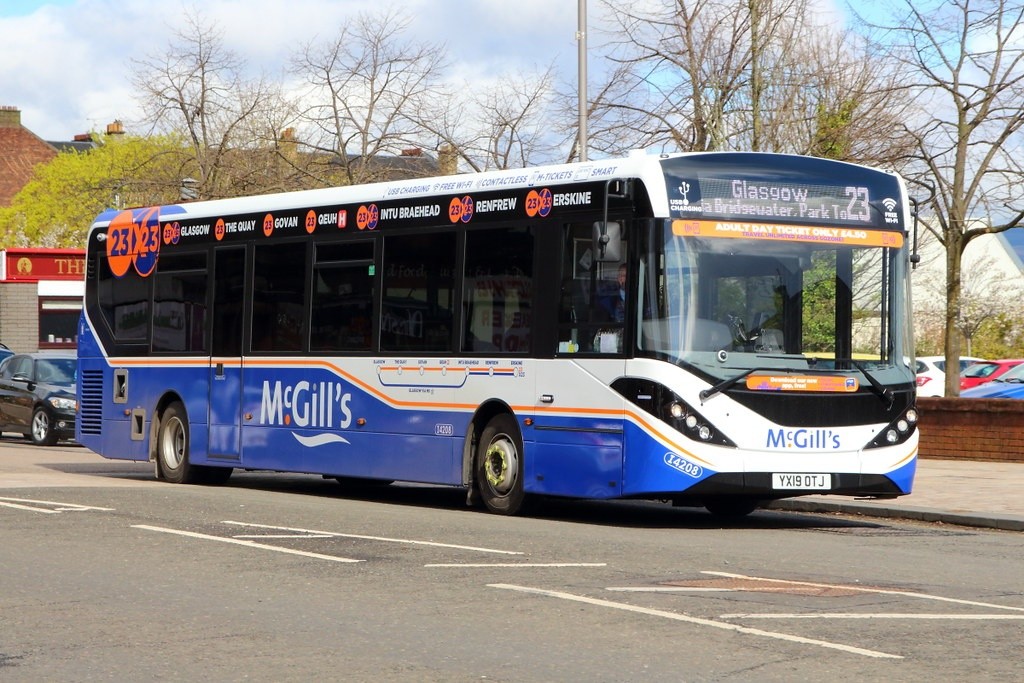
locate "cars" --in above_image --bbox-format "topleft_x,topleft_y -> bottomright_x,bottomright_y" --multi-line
911,355 -> 1021,399
0,353 -> 75,448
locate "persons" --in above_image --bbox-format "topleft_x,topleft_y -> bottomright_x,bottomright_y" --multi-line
606,263 -> 647,333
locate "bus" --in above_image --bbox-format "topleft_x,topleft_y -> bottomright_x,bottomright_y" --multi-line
75,151 -> 919,516
